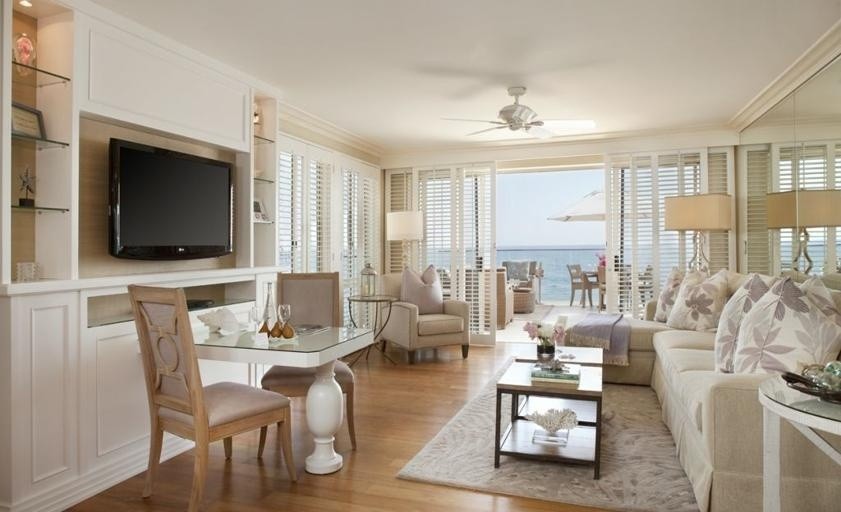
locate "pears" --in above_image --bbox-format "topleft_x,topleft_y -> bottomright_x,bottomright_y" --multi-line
282,318 -> 293,339
270,321 -> 282,338
258,318 -> 271,338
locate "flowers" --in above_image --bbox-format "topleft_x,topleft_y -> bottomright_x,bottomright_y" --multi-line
595,252 -> 606,267
523,319 -> 567,345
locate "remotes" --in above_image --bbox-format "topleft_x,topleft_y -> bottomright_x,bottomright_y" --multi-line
184,299 -> 215,310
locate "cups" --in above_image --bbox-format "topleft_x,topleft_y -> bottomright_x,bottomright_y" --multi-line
16,261 -> 42,283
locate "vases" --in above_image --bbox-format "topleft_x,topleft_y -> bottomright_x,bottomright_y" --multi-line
598,267 -> 606,284
536,338 -> 555,361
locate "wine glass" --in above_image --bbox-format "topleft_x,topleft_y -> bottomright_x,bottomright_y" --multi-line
252,306 -> 263,333
276,303 -> 291,339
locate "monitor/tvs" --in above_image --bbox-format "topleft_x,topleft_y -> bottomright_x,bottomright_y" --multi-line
108,137 -> 233,260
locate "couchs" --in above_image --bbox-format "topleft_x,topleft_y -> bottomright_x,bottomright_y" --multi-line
652,278 -> 841,512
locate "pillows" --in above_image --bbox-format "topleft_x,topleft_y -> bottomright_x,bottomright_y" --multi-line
653,269 -> 841,376
400,264 -> 444,315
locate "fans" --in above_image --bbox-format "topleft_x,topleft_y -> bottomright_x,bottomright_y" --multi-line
441,87 -> 595,143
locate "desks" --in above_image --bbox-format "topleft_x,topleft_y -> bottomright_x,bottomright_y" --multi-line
600,282 -> 653,317
195,326 -> 374,476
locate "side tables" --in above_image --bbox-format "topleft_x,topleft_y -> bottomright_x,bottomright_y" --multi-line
348,295 -> 397,367
758,376 -> 841,512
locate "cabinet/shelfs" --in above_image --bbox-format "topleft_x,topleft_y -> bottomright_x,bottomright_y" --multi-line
0,266 -> 287,512
236,94 -> 276,268
1,0 -> 74,284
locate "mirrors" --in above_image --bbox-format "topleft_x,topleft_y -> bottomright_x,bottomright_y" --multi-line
739,55 -> 841,290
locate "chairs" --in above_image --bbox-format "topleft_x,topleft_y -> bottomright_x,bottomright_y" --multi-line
567,264 -> 593,307
541,300 -> 671,388
257,272 -> 357,459
457,267 -> 514,330
378,271 -> 471,364
580,272 -> 600,308
502,261 -> 542,306
129,285 -> 298,512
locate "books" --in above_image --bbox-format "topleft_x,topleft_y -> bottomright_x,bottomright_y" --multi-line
531,363 -> 581,385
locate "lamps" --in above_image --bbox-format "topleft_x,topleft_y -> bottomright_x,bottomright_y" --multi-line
767,191 -> 841,282
664,193 -> 732,276
385,210 -> 424,273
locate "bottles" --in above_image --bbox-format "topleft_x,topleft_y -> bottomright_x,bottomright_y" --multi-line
11,31 -> 36,77
262,282 -> 276,332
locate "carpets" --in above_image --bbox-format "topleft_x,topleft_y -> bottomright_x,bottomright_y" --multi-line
394,355 -> 700,512
